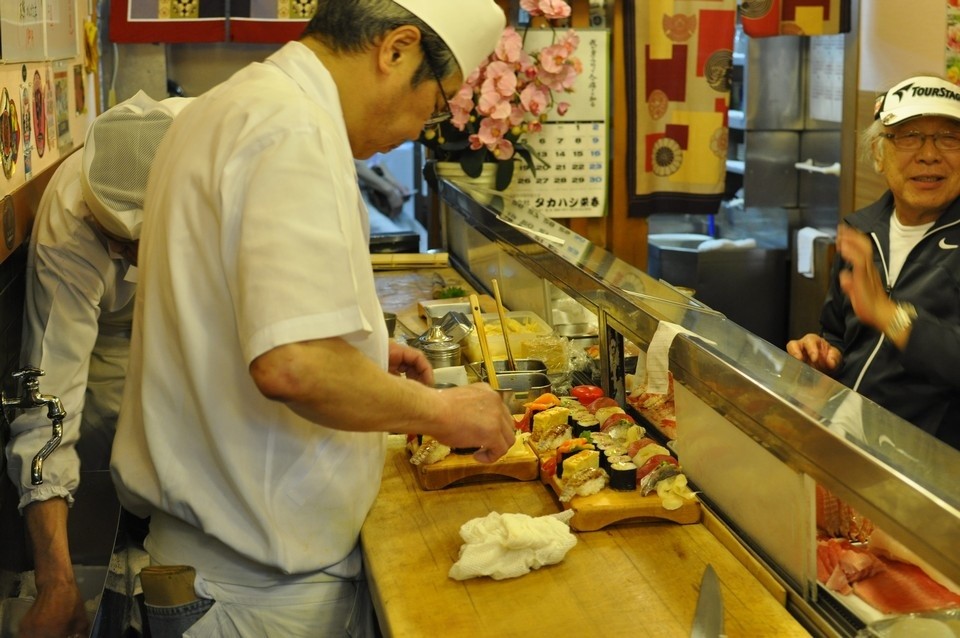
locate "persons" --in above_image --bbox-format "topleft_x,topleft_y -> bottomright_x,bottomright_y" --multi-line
786,75 -> 960,496
110,0 -> 518,638
5,87 -> 199,638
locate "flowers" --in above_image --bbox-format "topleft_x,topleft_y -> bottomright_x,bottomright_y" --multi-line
422,0 -> 583,192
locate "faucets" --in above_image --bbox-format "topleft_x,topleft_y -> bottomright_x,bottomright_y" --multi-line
683,213 -> 707,234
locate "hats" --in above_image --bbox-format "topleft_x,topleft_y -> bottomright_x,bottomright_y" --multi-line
873,75 -> 960,127
394,0 -> 506,83
80,88 -> 175,239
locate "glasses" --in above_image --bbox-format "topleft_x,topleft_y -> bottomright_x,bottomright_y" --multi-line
879,131 -> 960,150
391,24 -> 454,127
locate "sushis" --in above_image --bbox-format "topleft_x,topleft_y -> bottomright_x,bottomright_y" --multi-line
515,385 -> 702,511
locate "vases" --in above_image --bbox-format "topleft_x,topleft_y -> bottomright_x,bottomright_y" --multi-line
436,162 -> 497,206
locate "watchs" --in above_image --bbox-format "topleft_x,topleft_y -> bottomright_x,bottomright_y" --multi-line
887,302 -> 917,340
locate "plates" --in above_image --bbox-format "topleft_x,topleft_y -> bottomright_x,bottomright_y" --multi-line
816,541 -> 895,626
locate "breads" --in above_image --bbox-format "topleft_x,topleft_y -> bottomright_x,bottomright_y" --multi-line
521,338 -> 571,375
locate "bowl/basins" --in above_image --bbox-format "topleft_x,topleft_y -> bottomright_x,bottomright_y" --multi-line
461,311 -> 553,363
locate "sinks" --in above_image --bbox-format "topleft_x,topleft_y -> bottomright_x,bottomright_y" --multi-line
646,233 -> 715,282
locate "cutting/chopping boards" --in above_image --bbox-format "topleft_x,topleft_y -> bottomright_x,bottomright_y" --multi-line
359,434 -> 815,638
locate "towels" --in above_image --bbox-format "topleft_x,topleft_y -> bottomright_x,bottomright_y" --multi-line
797,226 -> 830,279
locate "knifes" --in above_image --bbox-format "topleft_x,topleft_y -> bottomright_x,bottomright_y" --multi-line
433,272 -> 445,299
689,563 -> 724,638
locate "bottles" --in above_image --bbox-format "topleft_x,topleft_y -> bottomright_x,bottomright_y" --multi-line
418,324 -> 461,369
